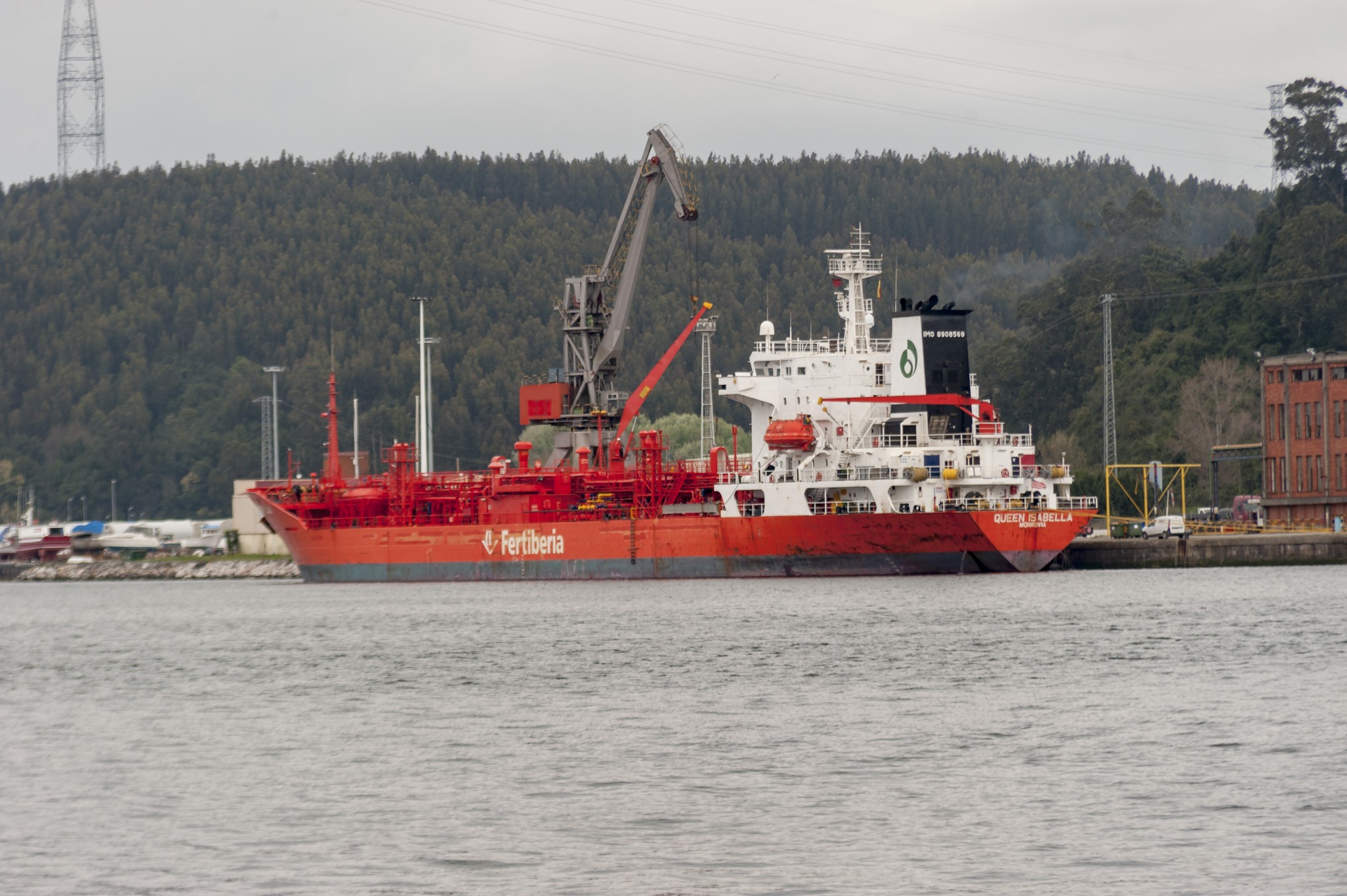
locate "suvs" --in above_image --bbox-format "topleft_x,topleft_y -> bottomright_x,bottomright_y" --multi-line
202,548 -> 222,557
1141,515 -> 1184,540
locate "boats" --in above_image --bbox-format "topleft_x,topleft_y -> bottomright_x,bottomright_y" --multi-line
764,416 -> 814,447
0,531 -> 225,555
244,129 -> 1098,582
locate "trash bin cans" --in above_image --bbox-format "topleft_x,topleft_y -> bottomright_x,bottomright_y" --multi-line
1125,523 -> 1142,538
1109,523 -> 1125,539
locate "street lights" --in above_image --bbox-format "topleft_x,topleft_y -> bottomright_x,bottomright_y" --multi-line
263,367 -> 286,478
416,337 -> 440,481
408,296 -> 432,482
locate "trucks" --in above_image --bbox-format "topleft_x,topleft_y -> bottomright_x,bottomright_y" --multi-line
1232,494 -> 1263,531
